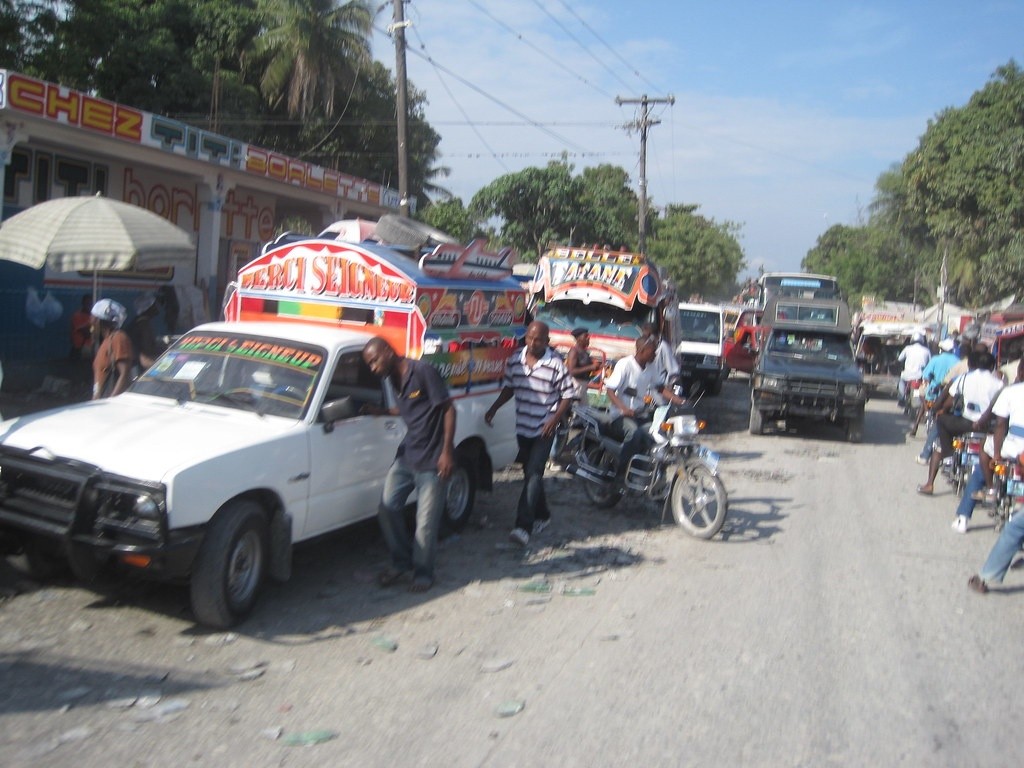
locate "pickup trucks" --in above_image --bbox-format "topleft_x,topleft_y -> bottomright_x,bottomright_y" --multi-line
527,248 -> 873,446
0,214 -> 533,633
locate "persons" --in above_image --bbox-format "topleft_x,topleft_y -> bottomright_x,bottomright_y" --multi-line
849,312 -> 882,373
898,331 -> 934,404
87,297 -> 137,400
642,323 -> 680,408
355,335 -> 457,593
950,354 -> 1024,594
545,327 -> 600,472
907,330 -> 1005,495
70,295 -> 96,360
603,334 -> 690,500
484,320 -> 575,547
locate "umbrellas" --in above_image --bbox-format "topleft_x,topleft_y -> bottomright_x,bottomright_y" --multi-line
0,188 -> 195,306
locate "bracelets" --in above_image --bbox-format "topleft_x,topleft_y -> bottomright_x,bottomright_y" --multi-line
681,399 -> 686,405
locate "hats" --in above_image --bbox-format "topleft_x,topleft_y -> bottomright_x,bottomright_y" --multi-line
134,292 -> 155,316
938,338 -> 954,352
571,328 -> 589,335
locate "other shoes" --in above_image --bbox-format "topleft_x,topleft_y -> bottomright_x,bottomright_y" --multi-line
610,485 -> 629,495
917,484 -> 933,497
910,432 -> 916,438
942,456 -> 953,466
532,516 -> 550,535
971,489 -> 997,503
915,454 -> 927,465
968,575 -> 987,593
509,528 -> 530,545
951,514 -> 968,534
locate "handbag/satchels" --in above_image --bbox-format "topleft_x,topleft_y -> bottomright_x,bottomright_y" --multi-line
952,373 -> 967,416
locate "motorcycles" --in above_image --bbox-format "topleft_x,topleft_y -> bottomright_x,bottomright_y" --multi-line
896,364 -> 1024,532
554,383 -> 728,539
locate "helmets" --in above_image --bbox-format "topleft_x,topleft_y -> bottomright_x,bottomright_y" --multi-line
910,332 -> 925,345
